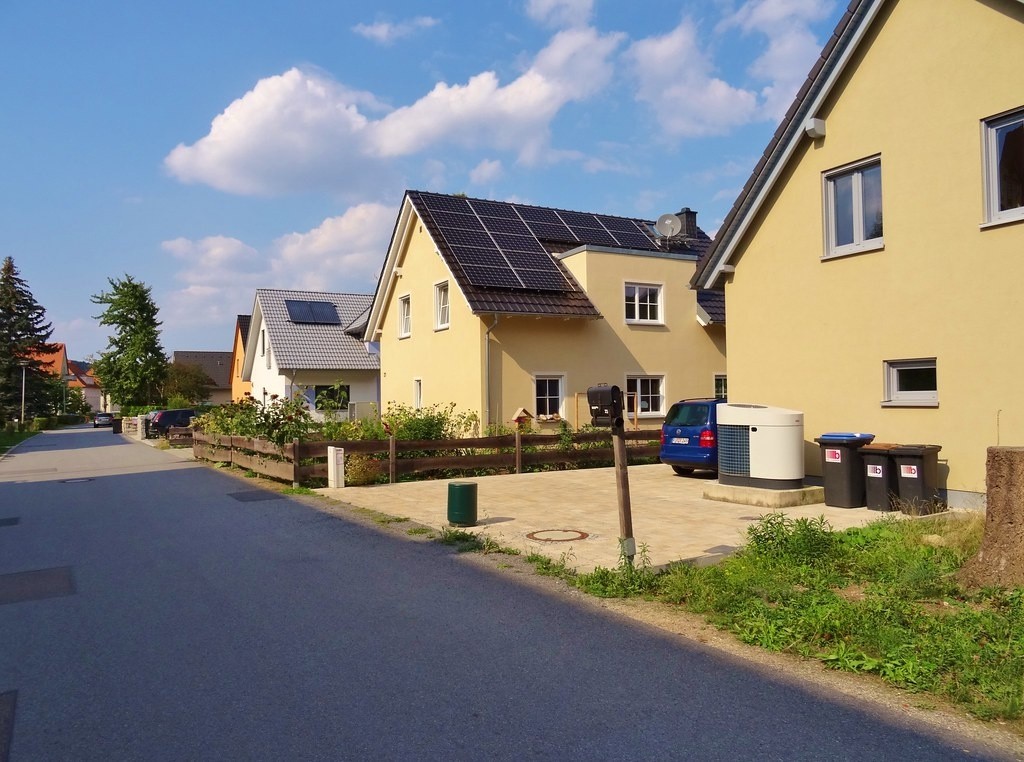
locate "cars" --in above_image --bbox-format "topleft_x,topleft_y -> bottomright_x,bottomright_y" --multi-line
93,412 -> 115,428
145,410 -> 195,439
658,397 -> 727,476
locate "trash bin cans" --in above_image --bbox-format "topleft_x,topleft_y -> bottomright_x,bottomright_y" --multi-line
813,431 -> 876,508
447,481 -> 479,527
858,443 -> 902,512
889,442 -> 944,516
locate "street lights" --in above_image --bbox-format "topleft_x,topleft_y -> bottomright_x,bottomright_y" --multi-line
17,360 -> 30,423
60,379 -> 68,415
